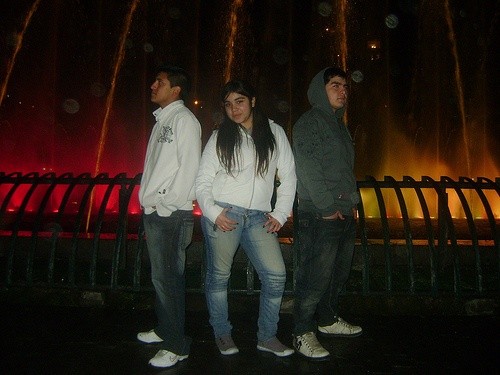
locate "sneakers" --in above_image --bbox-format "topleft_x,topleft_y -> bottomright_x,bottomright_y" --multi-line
147,349 -> 189,368
316,317 -> 364,339
136,329 -> 165,343
292,332 -> 331,362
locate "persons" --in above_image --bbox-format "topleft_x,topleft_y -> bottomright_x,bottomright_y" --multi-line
196,80 -> 297,357
138,64 -> 202,367
293,66 -> 363,358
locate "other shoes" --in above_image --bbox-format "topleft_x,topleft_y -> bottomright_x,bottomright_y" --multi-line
256,337 -> 295,356
215,334 -> 240,355
